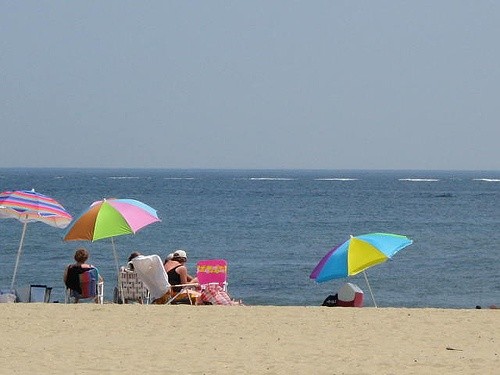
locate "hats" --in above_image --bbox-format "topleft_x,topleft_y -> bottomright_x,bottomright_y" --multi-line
166,253 -> 174,261
173,250 -> 187,258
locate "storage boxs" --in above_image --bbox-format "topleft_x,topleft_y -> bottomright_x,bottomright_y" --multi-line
29,284 -> 52,303
336,283 -> 364,306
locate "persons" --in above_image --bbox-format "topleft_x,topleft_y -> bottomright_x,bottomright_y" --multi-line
113,252 -> 139,304
64,249 -> 103,302
164,250 -> 200,295
164,254 -> 193,282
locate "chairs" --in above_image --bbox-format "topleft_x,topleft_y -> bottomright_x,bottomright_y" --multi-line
116,261 -> 149,305
65,267 -> 103,304
196,260 -> 228,303
133,255 -> 199,306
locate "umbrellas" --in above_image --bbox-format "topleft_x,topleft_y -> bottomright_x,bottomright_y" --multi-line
309,233 -> 413,308
61,198 -> 162,304
1,188 -> 72,295
